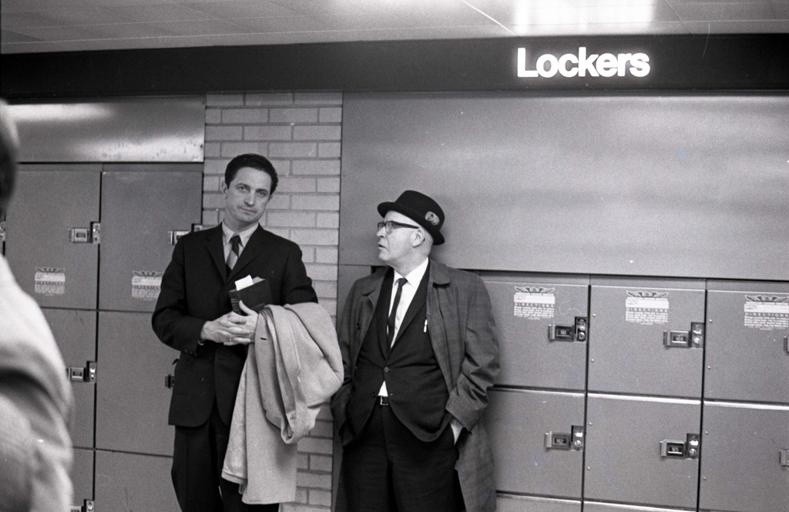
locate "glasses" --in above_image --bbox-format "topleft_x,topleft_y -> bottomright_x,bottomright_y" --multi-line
377,221 -> 425,242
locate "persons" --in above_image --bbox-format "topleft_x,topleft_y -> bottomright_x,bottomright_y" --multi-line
332,190 -> 500,512
0,101 -> 75,511
151,154 -> 318,512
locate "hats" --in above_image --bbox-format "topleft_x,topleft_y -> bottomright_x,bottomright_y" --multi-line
378,190 -> 446,246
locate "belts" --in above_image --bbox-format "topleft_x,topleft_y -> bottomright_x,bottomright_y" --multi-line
375,395 -> 391,406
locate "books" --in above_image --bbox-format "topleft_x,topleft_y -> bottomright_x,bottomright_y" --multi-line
228,273 -> 272,317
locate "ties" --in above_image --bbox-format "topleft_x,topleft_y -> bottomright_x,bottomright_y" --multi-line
388,278 -> 407,346
226,233 -> 242,280
